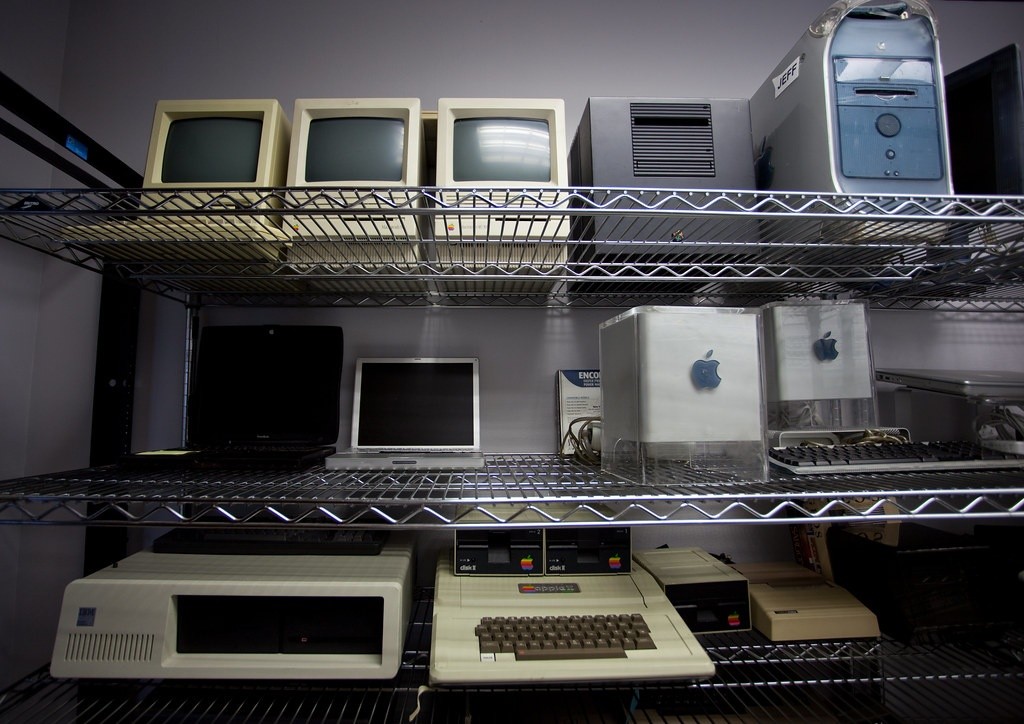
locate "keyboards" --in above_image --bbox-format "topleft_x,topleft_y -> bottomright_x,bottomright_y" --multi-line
767,439 -> 1024,472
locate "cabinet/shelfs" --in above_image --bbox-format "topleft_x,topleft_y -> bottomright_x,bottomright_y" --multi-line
0,186 -> 1024,530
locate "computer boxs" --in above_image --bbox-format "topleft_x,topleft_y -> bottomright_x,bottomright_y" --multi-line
749,0 -> 953,267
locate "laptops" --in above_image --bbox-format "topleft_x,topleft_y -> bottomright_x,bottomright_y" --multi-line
125,325 -> 343,473
874,367 -> 1024,399
325,356 -> 485,473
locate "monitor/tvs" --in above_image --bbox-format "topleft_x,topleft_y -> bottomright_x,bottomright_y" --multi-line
137,101 -> 571,295
